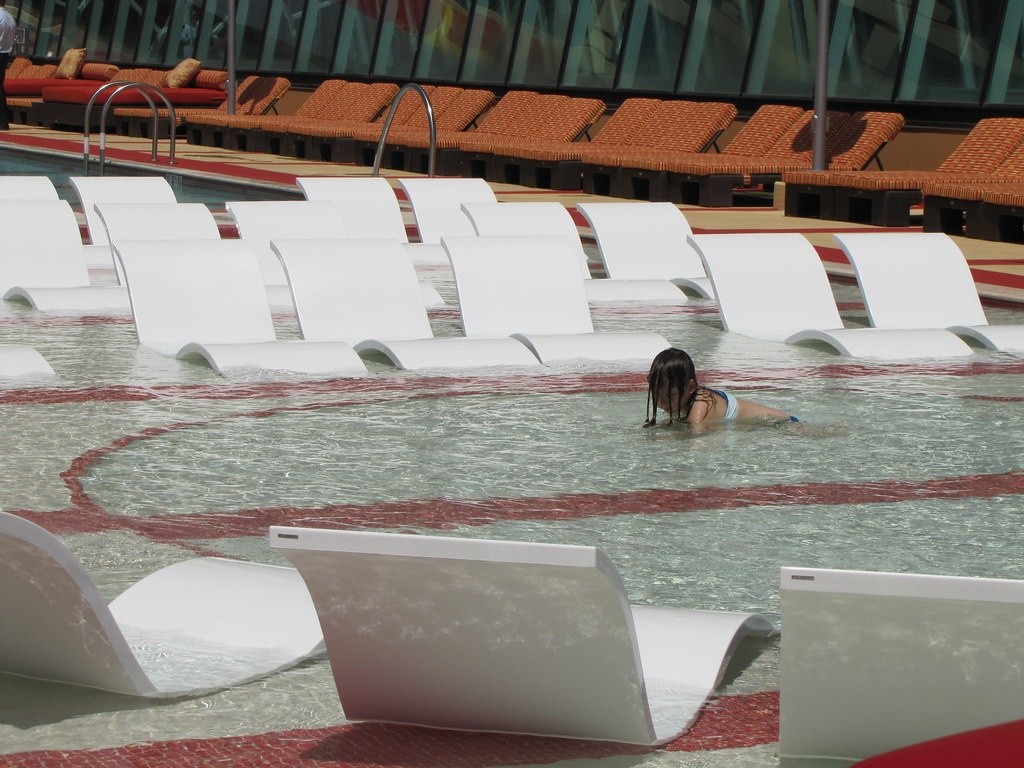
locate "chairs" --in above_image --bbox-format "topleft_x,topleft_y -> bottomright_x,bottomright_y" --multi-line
0,512 -> 326,698
2,174 -> 715,315
269,239 -> 541,372
441,238 -> 675,365
781,116 -> 1024,225
834,229 -> 1024,352
112,240 -> 370,377
777,565 -> 1024,768
3,59 -> 904,174
683,231 -> 974,366
265,524 -> 777,746
922,139 -> 1024,240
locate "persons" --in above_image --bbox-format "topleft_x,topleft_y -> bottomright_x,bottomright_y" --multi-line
644,347 -> 844,440
0,0 -> 18,130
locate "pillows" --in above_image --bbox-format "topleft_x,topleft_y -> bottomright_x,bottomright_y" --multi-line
18,65 -> 41,78
32,64 -> 58,78
165,58 -> 201,88
55,48 -> 88,80
192,69 -> 228,89
4,57 -> 33,79
79,63 -> 119,78
110,69 -> 168,88
